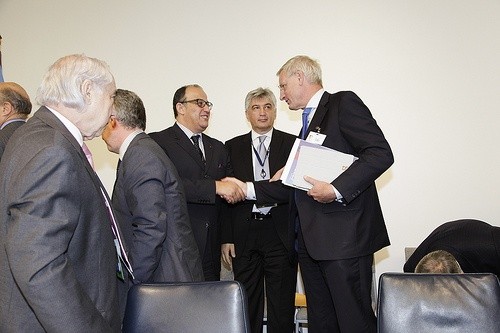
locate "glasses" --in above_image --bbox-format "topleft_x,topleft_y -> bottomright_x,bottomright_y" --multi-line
180,98 -> 214,110
277,72 -> 296,92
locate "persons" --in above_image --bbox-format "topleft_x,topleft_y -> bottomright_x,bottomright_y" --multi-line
0,82 -> 32,160
102,89 -> 205,284
147,84 -> 245,282
220,55 -> 394,333
403,218 -> 500,282
0,52 -> 135,333
218,87 -> 299,333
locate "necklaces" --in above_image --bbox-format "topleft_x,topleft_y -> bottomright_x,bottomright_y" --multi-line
251,142 -> 271,179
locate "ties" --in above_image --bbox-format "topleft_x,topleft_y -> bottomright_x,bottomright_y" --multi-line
190,134 -> 207,169
301,107 -> 313,141
82,140 -> 134,272
116,157 -> 122,179
254,134 -> 270,182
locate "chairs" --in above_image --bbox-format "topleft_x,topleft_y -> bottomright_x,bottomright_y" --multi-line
375,271 -> 500,333
124,278 -> 251,333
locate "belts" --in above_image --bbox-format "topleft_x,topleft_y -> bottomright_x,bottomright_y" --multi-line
250,210 -> 273,220
